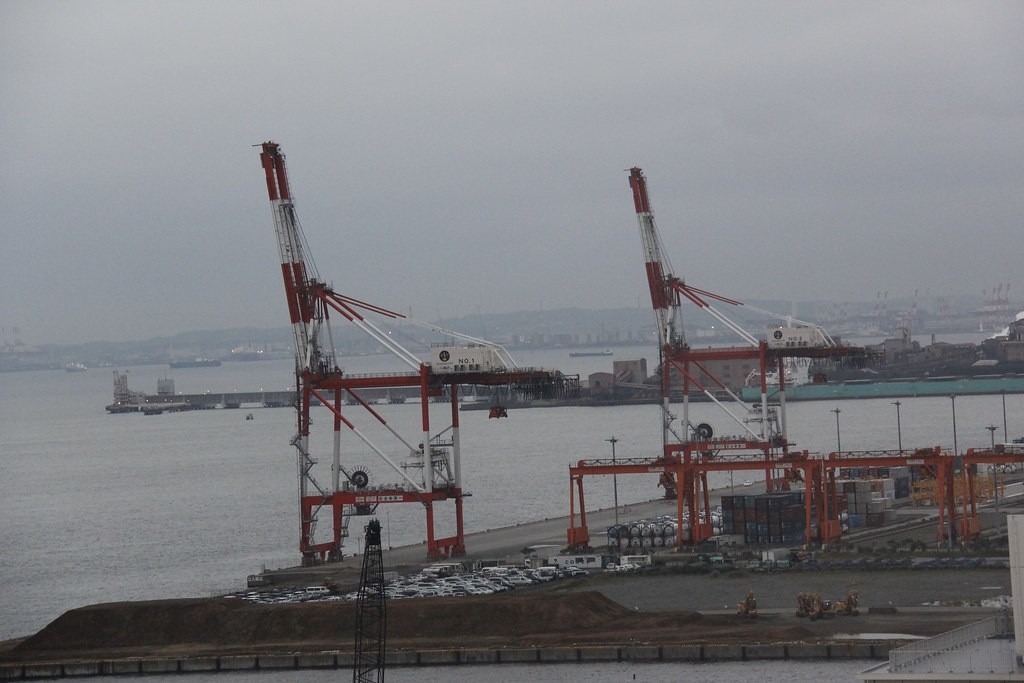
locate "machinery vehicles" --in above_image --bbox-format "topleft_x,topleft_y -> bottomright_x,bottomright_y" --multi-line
795,592 -> 860,621
737,591 -> 757,617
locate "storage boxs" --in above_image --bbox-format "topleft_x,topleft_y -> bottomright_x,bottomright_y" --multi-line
721,443 -> 1024,543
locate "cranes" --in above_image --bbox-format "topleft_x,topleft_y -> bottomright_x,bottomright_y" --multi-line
260,142 -> 581,568
910,290 -> 917,316
353,519 -> 386,683
978,284 -> 1010,312
628,167 -> 860,522
872,291 -> 887,315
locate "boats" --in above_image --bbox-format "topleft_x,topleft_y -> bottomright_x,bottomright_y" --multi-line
569,350 -> 614,357
66,363 -> 87,372
169,359 -> 221,368
742,314 -> 1024,402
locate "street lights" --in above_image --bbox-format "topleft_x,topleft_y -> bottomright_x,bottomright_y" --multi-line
830,408 -> 841,459
1000,389 -> 1007,443
891,400 -> 902,457
985,427 -> 998,512
605,437 -> 620,548
950,392 -> 957,456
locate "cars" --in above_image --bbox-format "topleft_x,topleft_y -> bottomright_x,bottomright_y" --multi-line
605,563 -> 640,572
743,481 -> 753,487
223,558 -> 588,603
